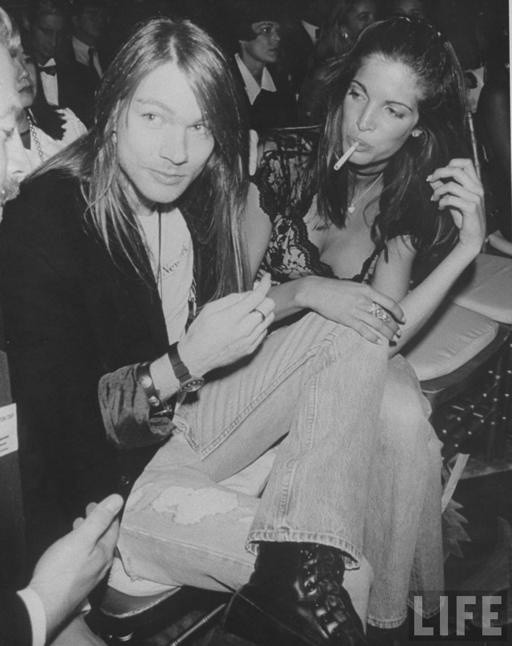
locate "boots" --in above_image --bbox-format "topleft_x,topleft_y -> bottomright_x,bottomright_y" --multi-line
222,542 -> 365,646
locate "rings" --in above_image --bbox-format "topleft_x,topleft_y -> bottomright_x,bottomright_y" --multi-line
369,304 -> 390,321
249,307 -> 267,321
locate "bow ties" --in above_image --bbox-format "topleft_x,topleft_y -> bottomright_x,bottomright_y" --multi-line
36,63 -> 57,78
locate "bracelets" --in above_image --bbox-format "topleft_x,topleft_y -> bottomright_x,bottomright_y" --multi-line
136,360 -> 163,411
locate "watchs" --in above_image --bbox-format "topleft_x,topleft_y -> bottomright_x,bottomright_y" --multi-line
164,339 -> 205,395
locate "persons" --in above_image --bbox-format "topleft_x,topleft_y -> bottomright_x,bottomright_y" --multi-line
0,17 -> 428,646
0,489 -> 127,646
0,0 -> 510,267
238,15 -> 490,646
